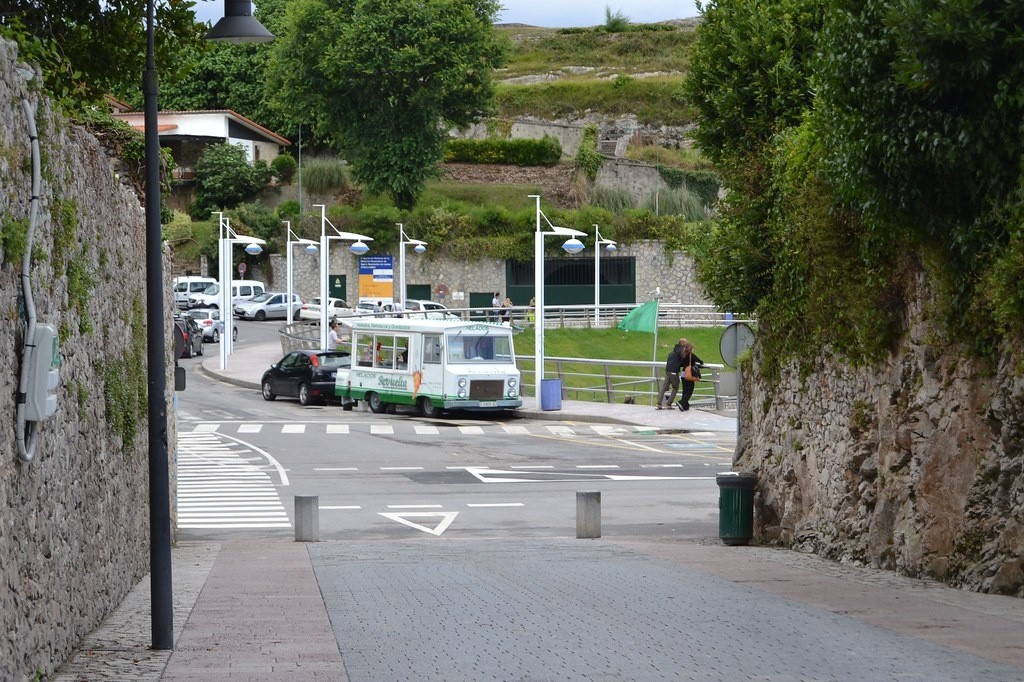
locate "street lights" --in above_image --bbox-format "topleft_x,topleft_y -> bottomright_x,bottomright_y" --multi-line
394,222 -> 429,317
528,194 -> 589,412
211,211 -> 265,372
312,204 -> 376,350
144,0 -> 276,643
591,223 -> 618,326
282,220 -> 321,324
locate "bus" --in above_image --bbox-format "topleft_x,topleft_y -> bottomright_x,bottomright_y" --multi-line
334,316 -> 522,419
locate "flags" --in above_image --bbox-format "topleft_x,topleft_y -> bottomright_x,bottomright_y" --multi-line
618,300 -> 657,332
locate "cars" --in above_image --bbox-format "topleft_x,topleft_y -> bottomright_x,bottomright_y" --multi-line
261,348 -> 352,406
186,308 -> 238,343
233,291 -> 303,321
300,296 -> 354,321
173,314 -> 205,358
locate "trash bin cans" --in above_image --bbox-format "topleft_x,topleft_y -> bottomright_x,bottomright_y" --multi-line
716,472 -> 757,545
541,378 -> 562,411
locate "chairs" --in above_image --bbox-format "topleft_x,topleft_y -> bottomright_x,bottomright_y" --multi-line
325,356 -> 334,364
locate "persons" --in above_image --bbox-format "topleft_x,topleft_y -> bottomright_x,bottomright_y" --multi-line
624,395 -> 634,404
492,292 -> 502,318
676,342 -> 704,412
526,297 -> 535,321
328,322 -> 346,351
397,340 -> 408,363
499,296 -> 513,322
655,338 -> 691,410
362,340 -> 384,364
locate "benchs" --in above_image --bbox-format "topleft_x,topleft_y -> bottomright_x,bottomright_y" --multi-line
358,361 -> 383,367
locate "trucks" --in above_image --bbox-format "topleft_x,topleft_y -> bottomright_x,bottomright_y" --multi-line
349,302 -> 386,319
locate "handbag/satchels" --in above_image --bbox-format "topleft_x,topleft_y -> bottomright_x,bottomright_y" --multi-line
684,365 -> 700,381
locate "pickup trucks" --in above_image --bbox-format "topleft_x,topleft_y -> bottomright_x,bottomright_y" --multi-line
384,299 -> 460,321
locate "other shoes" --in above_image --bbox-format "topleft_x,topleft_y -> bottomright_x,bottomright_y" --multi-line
657,404 -> 662,409
677,401 -> 684,411
666,405 -> 675,409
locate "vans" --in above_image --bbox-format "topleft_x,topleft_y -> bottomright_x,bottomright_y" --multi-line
187,280 -> 266,310
171,276 -> 218,310
